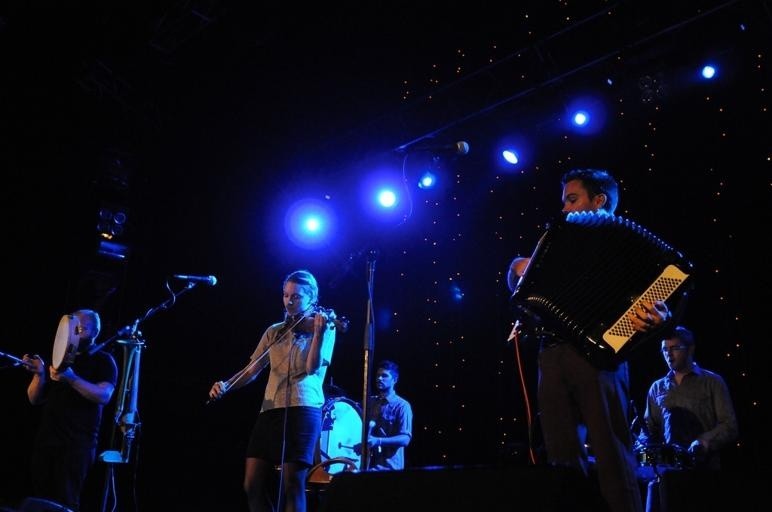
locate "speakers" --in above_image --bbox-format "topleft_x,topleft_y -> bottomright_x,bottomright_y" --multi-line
320,472 -> 563,512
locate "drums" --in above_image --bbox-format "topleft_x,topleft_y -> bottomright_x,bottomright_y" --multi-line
314,395 -> 372,478
51,314 -> 82,371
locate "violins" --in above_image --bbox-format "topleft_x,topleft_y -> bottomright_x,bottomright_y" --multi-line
290,305 -> 350,334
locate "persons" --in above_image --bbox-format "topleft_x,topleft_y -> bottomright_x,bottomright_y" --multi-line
633,325 -> 739,512
362,358 -> 415,472
504,164 -> 675,512
205,269 -> 339,512
16,305 -> 118,512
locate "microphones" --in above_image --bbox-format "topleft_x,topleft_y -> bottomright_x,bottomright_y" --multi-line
173,274 -> 217,286
420,140 -> 469,157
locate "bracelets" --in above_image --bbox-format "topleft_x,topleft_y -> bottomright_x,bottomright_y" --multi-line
380,438 -> 382,445
